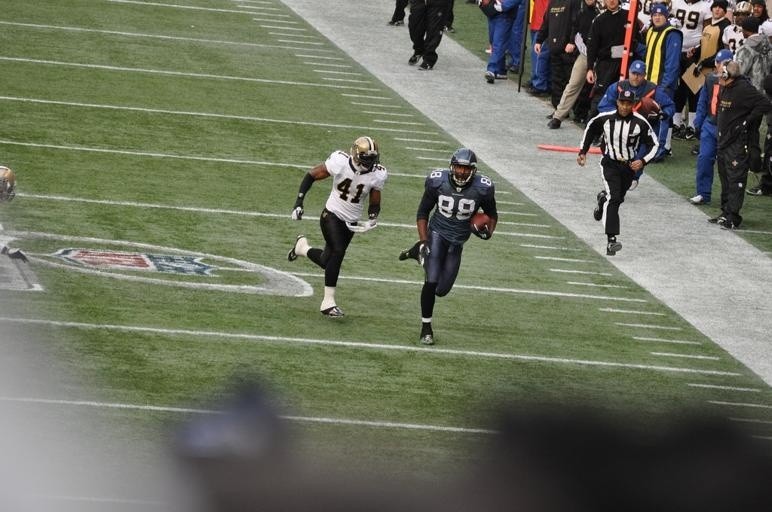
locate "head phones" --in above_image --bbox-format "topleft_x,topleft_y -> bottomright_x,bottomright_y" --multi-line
722,59 -> 732,80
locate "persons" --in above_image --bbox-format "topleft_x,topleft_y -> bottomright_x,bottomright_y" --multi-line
0,165 -> 30,264
287,135 -> 389,320
399,147 -> 500,347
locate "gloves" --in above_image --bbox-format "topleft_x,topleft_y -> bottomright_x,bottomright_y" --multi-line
470,223 -> 491,240
7,248 -> 29,263
291,206 -> 304,220
418,240 -> 430,265
368,212 -> 377,226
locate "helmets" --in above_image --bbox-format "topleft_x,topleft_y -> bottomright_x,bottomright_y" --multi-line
351,136 -> 379,169
733,0 -> 754,15
0,166 -> 17,201
448,148 -> 478,189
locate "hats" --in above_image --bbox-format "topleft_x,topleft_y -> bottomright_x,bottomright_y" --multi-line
652,3 -> 668,16
716,49 -> 733,61
618,90 -> 635,103
631,59 -> 645,75
712,0 -> 728,13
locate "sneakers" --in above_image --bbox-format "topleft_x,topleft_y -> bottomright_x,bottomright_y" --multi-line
746,187 -> 763,195
418,61 -> 431,70
690,194 -> 704,203
522,80 -> 585,128
287,235 -> 306,261
399,240 -> 421,260
708,213 -> 727,224
720,219 -> 743,230
673,123 -> 700,155
594,191 -> 607,220
420,328 -> 433,344
485,64 -> 519,84
320,300 -> 344,318
444,25 -> 454,32
607,242 -> 622,255
408,54 -> 422,64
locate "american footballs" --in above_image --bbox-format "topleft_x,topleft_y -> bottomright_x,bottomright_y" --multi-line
469,212 -> 490,234
641,97 -> 659,113
